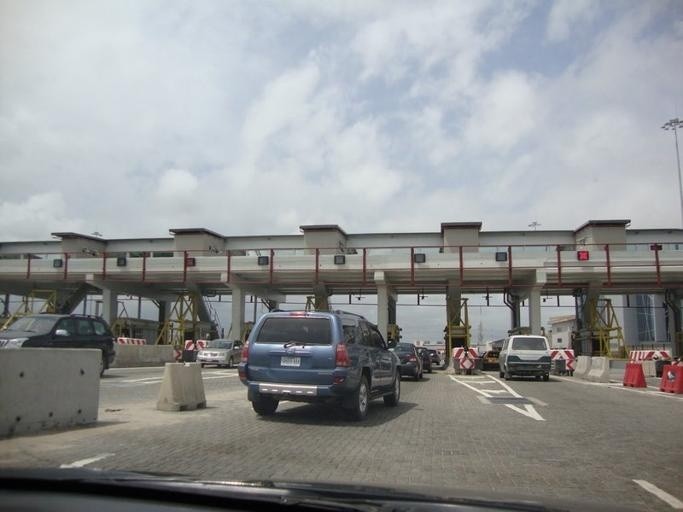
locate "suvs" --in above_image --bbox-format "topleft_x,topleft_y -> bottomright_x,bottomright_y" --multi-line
236,309 -> 400,420
0,312 -> 116,377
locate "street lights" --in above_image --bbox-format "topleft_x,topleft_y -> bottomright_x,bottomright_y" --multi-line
660,118 -> 683,225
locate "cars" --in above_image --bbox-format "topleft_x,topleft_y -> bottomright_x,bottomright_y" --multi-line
196,339 -> 244,368
391,343 -> 422,379
429,351 -> 442,366
498,336 -> 552,382
483,349 -> 499,368
415,346 -> 432,374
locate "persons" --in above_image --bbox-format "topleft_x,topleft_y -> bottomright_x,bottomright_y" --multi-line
654,355 -> 682,377
